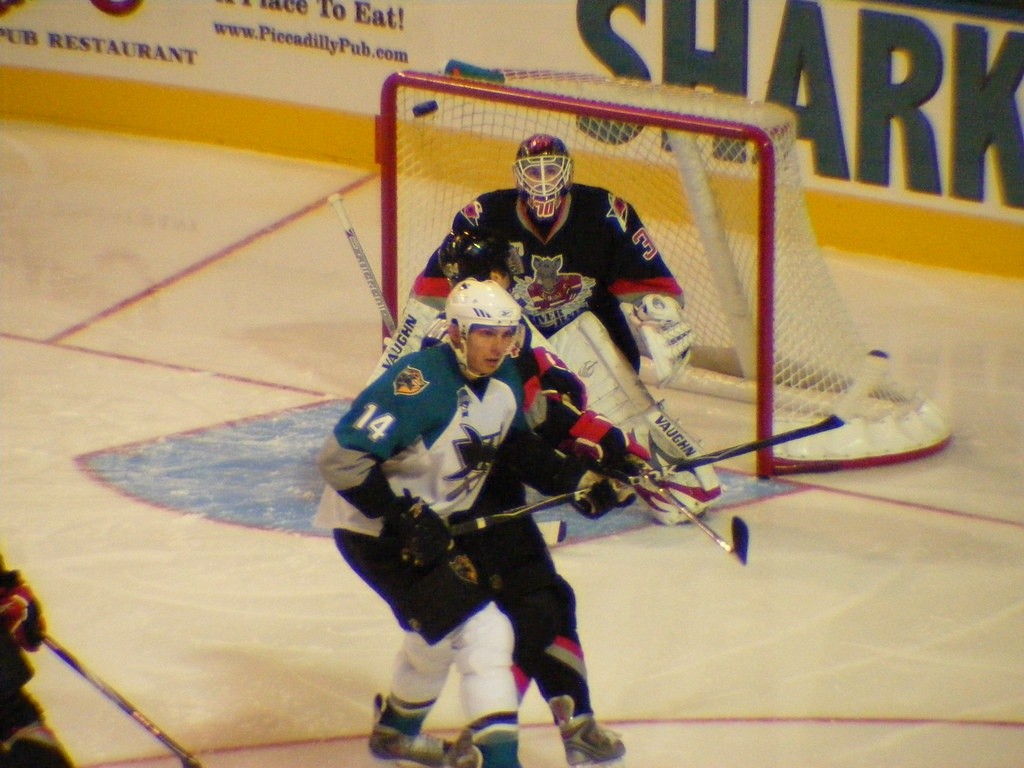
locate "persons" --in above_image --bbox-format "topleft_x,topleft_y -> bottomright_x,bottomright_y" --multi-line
0,554 -> 81,768
317,276 -> 653,768
375,133 -> 724,527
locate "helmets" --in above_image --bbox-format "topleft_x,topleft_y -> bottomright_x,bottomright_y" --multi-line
438,227 -> 516,282
514,133 -> 575,219
444,275 -> 521,345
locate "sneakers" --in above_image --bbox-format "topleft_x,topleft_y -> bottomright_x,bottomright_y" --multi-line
368,691 -> 453,768
550,694 -> 626,768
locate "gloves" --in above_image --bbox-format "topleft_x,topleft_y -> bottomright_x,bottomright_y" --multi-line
384,498 -> 455,564
565,452 -> 637,518
0,570 -> 46,653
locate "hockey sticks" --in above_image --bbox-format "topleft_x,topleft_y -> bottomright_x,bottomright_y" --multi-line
41,630 -> 203,767
327,191 -> 569,548
656,486 -> 751,567
445,346 -> 894,539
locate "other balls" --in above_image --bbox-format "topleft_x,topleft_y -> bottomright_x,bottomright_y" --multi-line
413,99 -> 439,118
